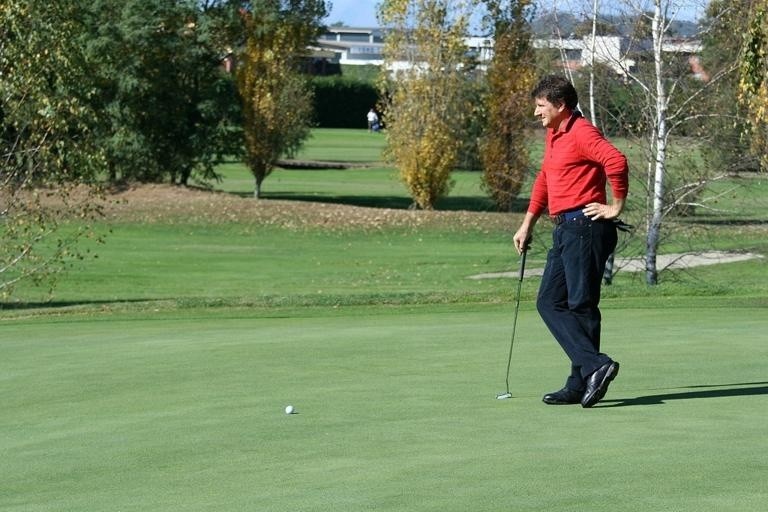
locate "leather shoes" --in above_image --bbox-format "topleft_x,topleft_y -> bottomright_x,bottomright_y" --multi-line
580,358 -> 621,409
542,386 -> 586,405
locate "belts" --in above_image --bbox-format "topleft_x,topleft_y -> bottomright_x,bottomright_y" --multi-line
553,204 -> 590,225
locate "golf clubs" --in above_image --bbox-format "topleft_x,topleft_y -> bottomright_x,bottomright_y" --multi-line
496,240 -> 528,399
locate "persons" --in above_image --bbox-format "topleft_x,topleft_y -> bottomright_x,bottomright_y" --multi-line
367,109 -> 378,132
513,70 -> 630,408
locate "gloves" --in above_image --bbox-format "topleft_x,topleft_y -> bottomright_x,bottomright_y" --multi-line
613,218 -> 635,234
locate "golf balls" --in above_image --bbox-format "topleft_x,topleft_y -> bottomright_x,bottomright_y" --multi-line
285,405 -> 295,414
